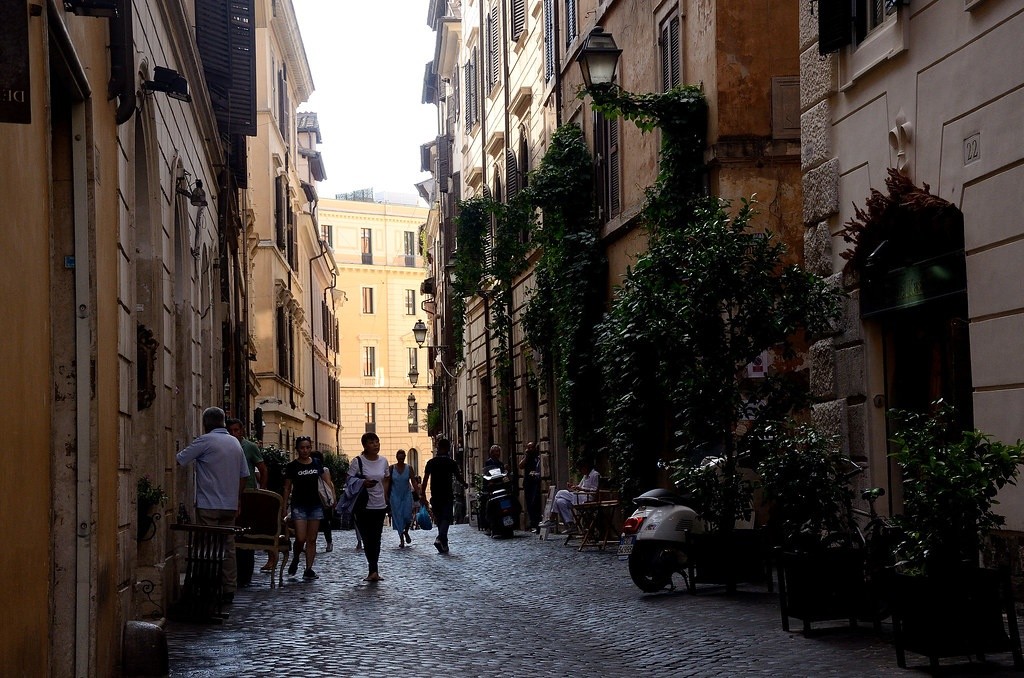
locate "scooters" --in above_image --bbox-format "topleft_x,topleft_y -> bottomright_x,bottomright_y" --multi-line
615,454 -> 730,594
472,468 -> 516,540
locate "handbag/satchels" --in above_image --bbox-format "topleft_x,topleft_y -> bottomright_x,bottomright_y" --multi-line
353,457 -> 370,512
387,464 -> 394,495
314,458 -> 335,507
416,504 -> 432,530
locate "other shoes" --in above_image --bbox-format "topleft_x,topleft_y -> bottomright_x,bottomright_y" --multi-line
434,539 -> 449,554
538,520 -> 555,527
288,557 -> 299,576
364,572 -> 385,582
357,544 -> 361,549
562,527 -> 580,534
302,569 -> 319,579
404,531 -> 411,543
261,563 -> 273,570
399,544 -> 404,548
326,542 -> 333,552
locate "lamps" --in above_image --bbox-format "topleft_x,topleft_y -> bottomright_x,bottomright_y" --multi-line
140,64 -> 194,114
173,180 -> 207,208
408,365 -> 437,389
408,393 -> 430,411
573,25 -> 696,115
413,318 -> 449,350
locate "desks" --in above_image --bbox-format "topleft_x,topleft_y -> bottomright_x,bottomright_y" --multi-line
578,498 -> 620,551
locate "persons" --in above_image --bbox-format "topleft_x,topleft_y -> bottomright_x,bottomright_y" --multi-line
538,458 -> 600,534
348,433 -> 390,581
177,406 -> 250,603
282,436 -> 332,581
422,440 -> 469,552
519,442 -> 541,536
410,475 -> 421,531
227,418 -> 268,584
389,450 -> 418,548
481,445 -> 508,535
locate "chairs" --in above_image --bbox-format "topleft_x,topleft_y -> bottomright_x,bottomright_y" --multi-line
234,490 -> 292,589
562,475 -> 616,545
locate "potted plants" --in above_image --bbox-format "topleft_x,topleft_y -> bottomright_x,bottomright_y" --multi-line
601,186 -> 850,606
890,397 -> 1024,677
136,478 -> 164,544
135,324 -> 160,411
762,423 -> 879,639
